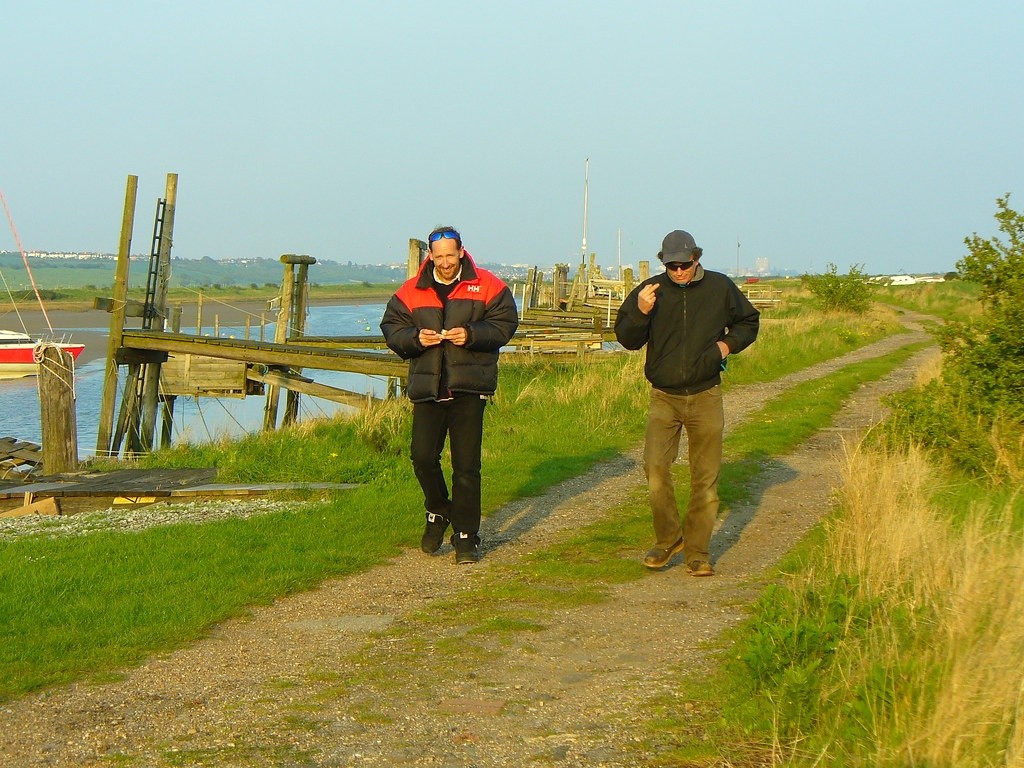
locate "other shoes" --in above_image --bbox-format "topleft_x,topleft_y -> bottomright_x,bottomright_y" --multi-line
422,511 -> 451,553
451,532 -> 481,564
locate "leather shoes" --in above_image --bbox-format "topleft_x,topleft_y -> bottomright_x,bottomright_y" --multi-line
686,561 -> 714,576
643,537 -> 684,568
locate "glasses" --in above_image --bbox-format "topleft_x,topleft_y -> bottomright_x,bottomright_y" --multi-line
429,231 -> 460,242
665,258 -> 695,271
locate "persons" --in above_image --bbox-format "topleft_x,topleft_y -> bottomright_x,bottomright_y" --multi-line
380,228 -> 519,564
613,230 -> 761,576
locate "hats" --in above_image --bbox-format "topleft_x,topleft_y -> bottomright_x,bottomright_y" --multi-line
662,230 -> 697,264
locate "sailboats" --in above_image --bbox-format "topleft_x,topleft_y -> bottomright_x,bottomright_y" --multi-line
0,191 -> 86,380
